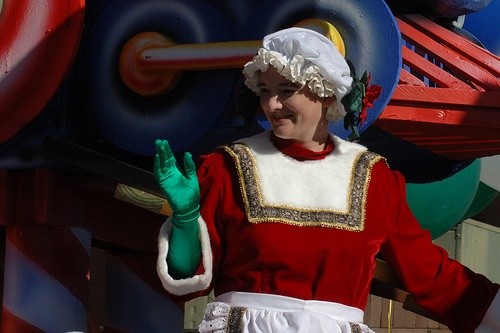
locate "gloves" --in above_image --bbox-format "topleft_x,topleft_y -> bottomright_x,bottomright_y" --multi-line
153,139 -> 200,280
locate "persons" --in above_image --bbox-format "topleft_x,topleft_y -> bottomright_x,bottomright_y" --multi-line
155,27 -> 500,333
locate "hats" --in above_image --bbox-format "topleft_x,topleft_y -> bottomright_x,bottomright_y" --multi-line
242,27 -> 352,122
